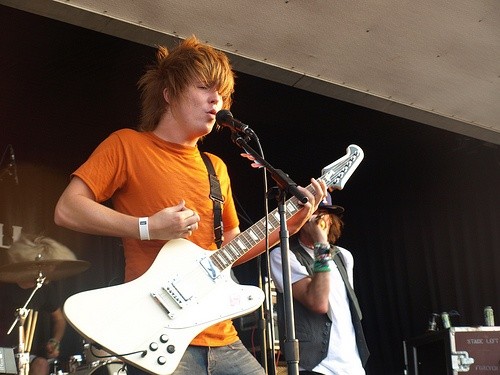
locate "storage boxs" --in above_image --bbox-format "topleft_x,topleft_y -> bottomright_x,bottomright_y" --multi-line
403,326 -> 500,375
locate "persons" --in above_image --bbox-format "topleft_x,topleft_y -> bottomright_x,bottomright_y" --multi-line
54,33 -> 265,375
0,232 -> 67,375
269,192 -> 365,375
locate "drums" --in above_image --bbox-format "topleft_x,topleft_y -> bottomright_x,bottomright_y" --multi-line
83,344 -> 118,362
71,359 -> 148,375
69,354 -> 83,373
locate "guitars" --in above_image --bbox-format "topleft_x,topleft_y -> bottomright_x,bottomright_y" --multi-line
63,144 -> 365,375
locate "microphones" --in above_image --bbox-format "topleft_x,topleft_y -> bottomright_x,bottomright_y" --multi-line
215,109 -> 255,135
316,215 -> 326,230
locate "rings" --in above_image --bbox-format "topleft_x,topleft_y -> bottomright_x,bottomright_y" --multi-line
188,225 -> 192,231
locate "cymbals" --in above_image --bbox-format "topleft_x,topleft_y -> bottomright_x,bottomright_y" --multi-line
0,259 -> 90,282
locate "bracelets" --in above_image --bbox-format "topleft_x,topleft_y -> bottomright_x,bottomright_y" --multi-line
138,217 -> 150,240
313,242 -> 340,272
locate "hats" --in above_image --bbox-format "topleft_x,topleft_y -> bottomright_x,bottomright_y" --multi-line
318,192 -> 345,217
